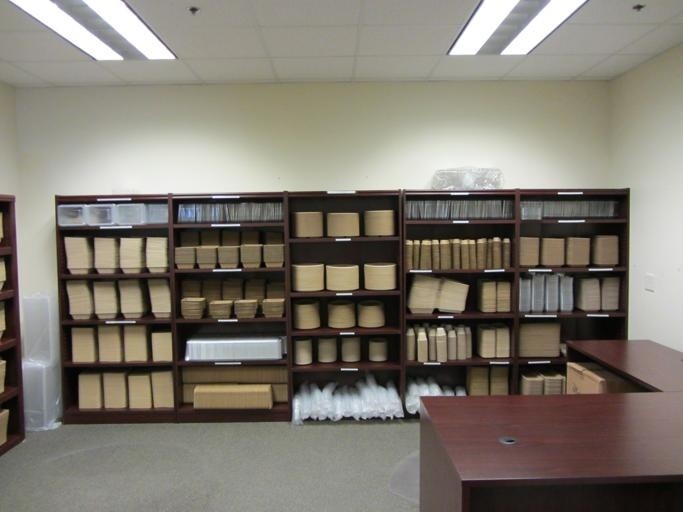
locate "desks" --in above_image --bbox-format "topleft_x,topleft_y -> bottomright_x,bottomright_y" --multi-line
419,340 -> 683,512
565,340 -> 683,392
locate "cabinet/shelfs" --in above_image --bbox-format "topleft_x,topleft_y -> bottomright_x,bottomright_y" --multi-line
0,194 -> 26,455
53,187 -> 631,424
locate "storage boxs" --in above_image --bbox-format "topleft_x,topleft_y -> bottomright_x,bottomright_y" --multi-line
116,203 -> 147,226
147,203 -> 168,225
566,361 -> 642,394
57,203 -> 86,228
86,203 -> 116,227
